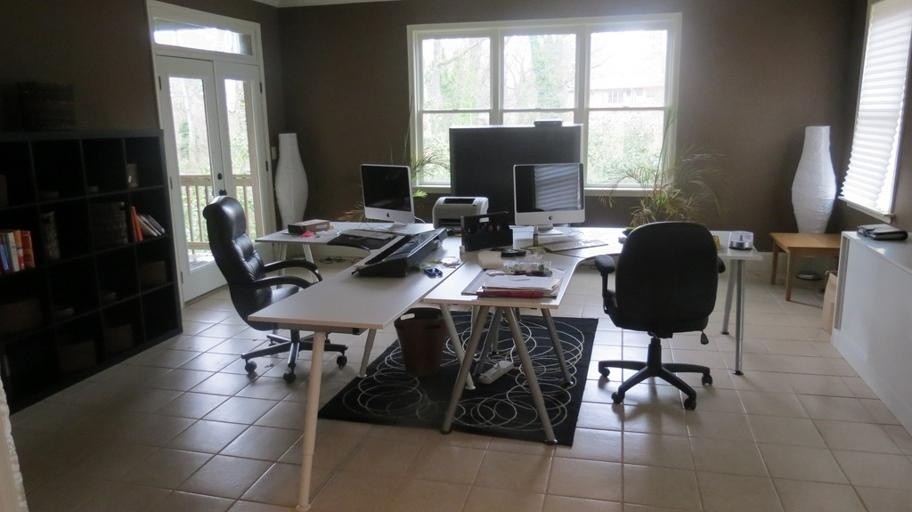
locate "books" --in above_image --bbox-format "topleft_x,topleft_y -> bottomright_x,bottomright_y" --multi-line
856,223 -> 908,241
0,199 -> 166,274
477,275 -> 562,298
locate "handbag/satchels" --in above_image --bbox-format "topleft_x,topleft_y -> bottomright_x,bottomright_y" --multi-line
88,200 -> 130,250
41,211 -> 59,261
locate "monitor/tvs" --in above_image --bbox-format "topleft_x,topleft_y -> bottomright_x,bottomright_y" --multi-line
513,162 -> 586,235
360,163 -> 415,231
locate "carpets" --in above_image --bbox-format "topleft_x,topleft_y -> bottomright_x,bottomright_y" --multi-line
316,311 -> 599,448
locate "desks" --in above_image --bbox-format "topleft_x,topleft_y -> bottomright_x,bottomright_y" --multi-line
423,227 -> 761,445
253,217 -> 471,512
771,232 -> 841,299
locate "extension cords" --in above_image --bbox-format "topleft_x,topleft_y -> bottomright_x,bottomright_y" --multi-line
479,360 -> 513,384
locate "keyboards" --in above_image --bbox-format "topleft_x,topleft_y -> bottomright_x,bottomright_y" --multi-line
340,228 -> 396,241
541,238 -> 610,252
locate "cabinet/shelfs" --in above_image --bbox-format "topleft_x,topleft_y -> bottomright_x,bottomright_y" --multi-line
1,126 -> 183,415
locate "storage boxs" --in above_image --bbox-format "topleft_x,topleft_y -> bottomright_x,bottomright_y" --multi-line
822,273 -> 838,334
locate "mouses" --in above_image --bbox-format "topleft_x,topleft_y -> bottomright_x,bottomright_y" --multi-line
618,235 -> 627,243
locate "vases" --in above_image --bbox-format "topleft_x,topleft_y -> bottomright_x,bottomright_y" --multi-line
276,131 -> 320,285
791,125 -> 839,231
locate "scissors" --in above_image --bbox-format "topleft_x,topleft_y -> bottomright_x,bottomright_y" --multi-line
423,266 -> 442,276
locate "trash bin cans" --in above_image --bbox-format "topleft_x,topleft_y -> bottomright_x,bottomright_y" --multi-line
393,307 -> 446,374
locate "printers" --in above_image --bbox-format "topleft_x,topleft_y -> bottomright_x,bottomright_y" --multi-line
432,195 -> 489,232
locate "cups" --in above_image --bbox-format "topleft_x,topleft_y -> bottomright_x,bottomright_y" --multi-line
126,164 -> 138,188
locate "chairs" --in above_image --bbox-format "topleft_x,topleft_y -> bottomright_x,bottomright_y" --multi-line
594,221 -> 718,411
202,196 -> 349,381
